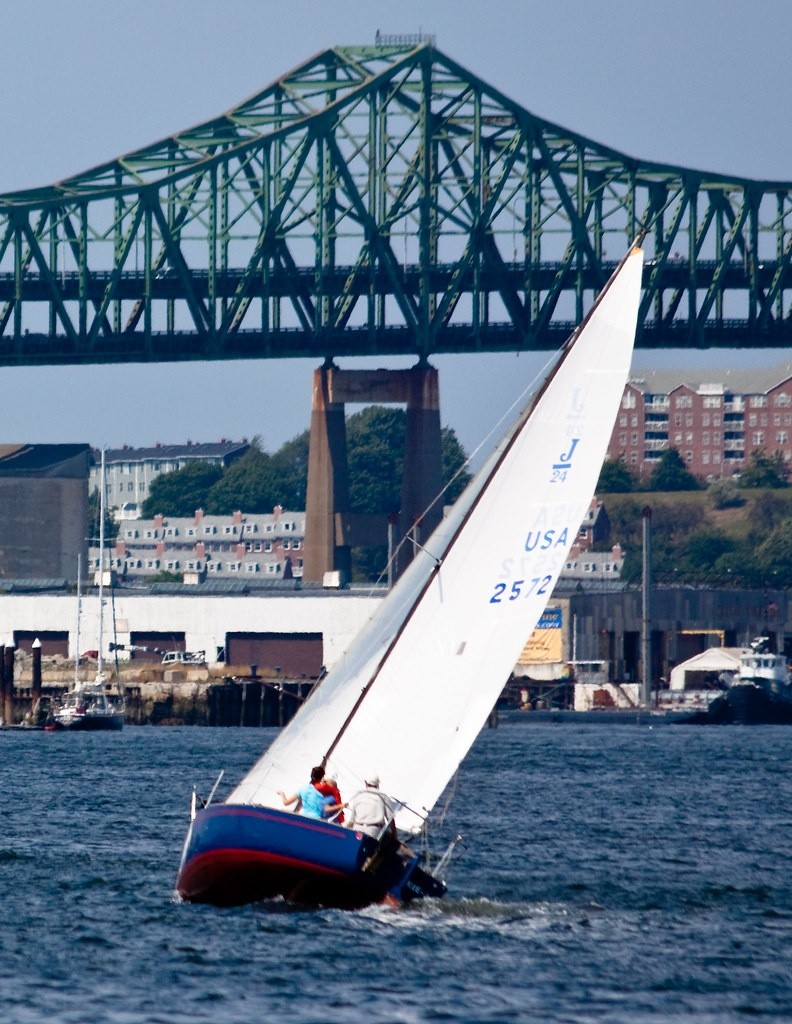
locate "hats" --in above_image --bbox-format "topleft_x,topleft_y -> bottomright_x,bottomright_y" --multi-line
364,774 -> 380,786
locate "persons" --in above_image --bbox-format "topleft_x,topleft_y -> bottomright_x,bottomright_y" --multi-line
344,774 -> 396,841
275,766 -> 349,824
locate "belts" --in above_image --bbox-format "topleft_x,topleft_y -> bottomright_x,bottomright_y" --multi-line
355,823 -> 383,828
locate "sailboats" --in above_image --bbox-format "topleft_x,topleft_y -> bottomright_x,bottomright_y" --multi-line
175,173 -> 682,916
51,442 -> 128,733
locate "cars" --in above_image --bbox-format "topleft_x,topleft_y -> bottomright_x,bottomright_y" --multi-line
161,650 -> 206,668
